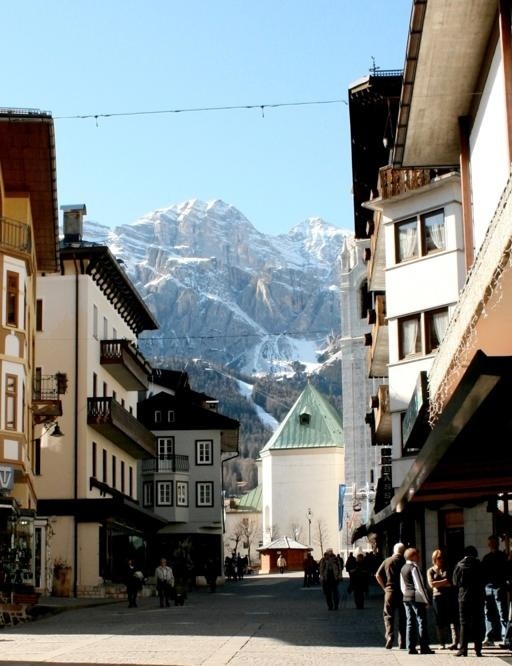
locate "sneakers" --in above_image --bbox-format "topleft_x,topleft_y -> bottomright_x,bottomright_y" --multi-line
477,651 -> 482,657
386,636 -> 435,655
439,645 -> 467,657
483,640 -> 495,646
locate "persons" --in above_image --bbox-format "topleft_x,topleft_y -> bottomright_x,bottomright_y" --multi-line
300,535 -> 512,658
277,555 -> 288,573
206,559 -> 219,593
154,557 -> 175,609
182,563 -> 194,593
224,551 -> 249,584
125,558 -> 145,610
